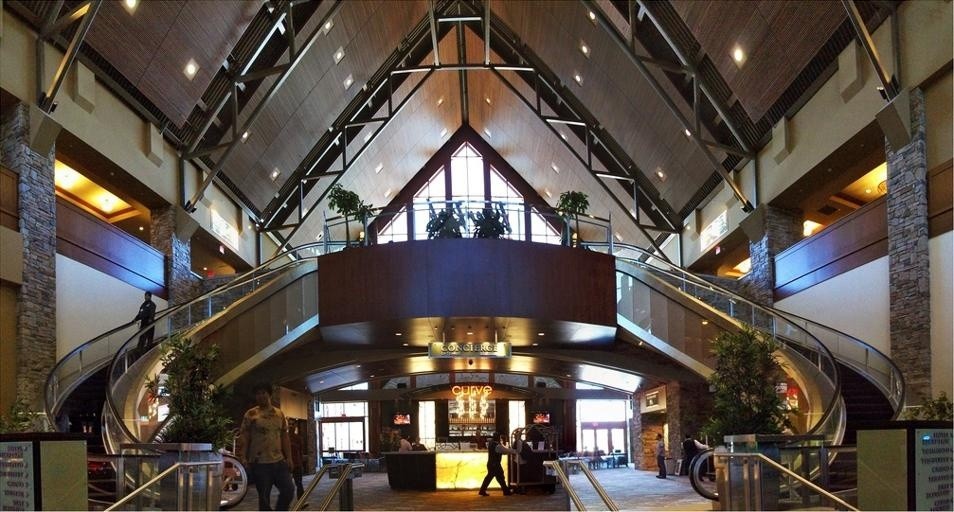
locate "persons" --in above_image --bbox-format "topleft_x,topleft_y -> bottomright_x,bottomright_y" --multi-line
240,385 -> 295,510
558,446 -> 601,470
478,432 -> 516,496
133,291 -> 156,351
399,434 -> 412,452
411,436 -> 426,450
288,415 -> 309,510
681,432 -> 709,482
513,431 -> 530,450
654,433 -> 666,479
470,428 -> 487,450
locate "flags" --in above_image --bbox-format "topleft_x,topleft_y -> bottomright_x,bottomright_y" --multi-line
321,213 -> 330,256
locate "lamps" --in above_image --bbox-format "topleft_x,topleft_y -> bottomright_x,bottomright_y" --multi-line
394,414 -> 411,425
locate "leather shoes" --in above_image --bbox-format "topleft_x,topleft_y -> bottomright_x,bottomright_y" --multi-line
656,475 -> 666,479
478,491 -> 490,496
507,489 -> 515,496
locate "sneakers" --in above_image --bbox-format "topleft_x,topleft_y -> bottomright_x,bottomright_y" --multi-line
300,501 -> 310,510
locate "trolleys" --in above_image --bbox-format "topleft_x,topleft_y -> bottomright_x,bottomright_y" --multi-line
509,425 -> 559,495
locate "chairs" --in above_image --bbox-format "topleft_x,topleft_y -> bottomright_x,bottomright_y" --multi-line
588,450 -> 628,470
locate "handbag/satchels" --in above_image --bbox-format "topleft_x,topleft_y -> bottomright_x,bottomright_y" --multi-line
519,442 -> 534,460
